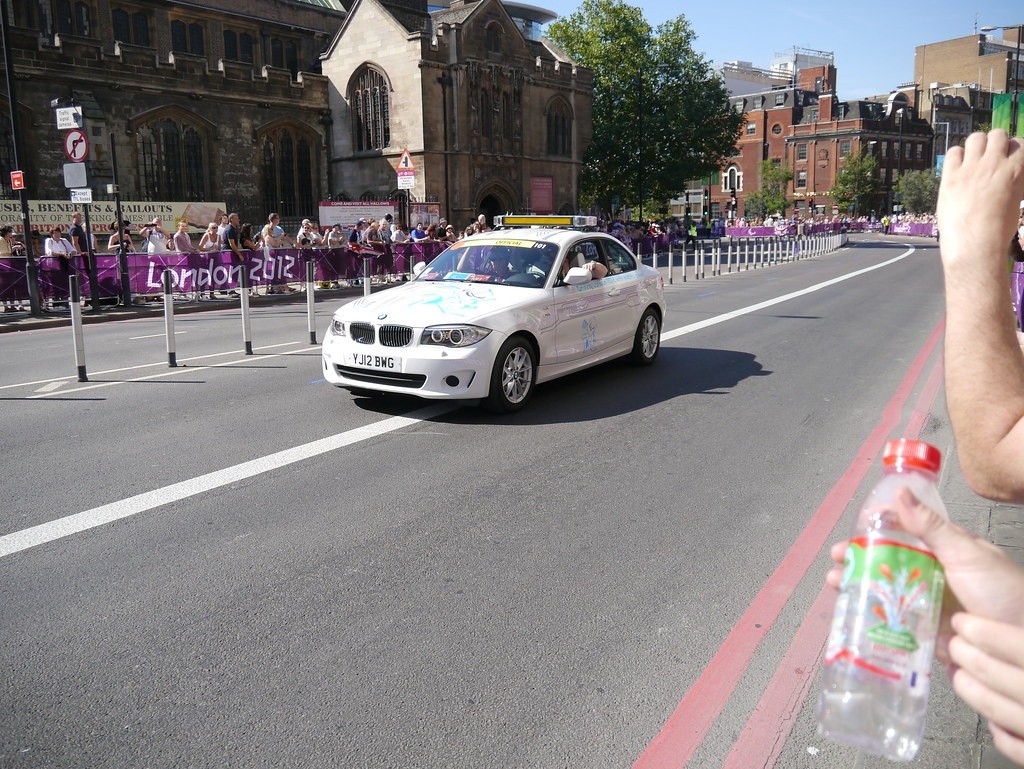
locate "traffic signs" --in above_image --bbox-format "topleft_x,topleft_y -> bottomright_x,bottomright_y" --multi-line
10,171 -> 25,191
71,188 -> 92,204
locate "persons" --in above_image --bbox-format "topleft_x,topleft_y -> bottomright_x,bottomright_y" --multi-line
821,484 -> 1024,767
0,209 -> 1024,313
935,129 -> 1024,506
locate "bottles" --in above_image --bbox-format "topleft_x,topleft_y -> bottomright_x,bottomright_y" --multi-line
815,438 -> 948,761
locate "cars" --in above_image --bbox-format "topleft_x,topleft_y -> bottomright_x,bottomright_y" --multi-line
321,215 -> 667,415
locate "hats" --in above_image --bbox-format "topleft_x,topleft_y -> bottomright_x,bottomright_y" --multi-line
356,220 -> 365,226
446,225 -> 455,233
440,217 -> 447,224
332,223 -> 340,227
359,218 -> 366,222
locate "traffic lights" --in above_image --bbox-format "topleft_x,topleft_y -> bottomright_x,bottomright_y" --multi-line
729,188 -> 735,197
686,207 -> 690,217
703,206 -> 708,215
732,198 -> 737,209
704,190 -> 708,200
808,198 -> 813,207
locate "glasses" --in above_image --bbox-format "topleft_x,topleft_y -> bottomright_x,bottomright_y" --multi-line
32,237 -> 37,239
305,226 -> 310,230
212,226 -> 218,229
448,228 -> 452,229
7,230 -> 12,233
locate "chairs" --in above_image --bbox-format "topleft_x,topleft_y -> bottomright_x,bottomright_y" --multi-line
568,251 -> 584,270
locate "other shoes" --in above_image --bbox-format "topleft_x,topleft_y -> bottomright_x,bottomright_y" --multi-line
2,286 -> 303,316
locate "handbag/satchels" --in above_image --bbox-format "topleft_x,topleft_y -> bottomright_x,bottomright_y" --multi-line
302,233 -> 310,245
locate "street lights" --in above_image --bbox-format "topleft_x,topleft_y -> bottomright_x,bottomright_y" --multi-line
980,25 -> 1022,137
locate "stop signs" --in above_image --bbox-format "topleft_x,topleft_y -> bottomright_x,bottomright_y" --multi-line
794,207 -> 800,213
832,205 -> 838,210
